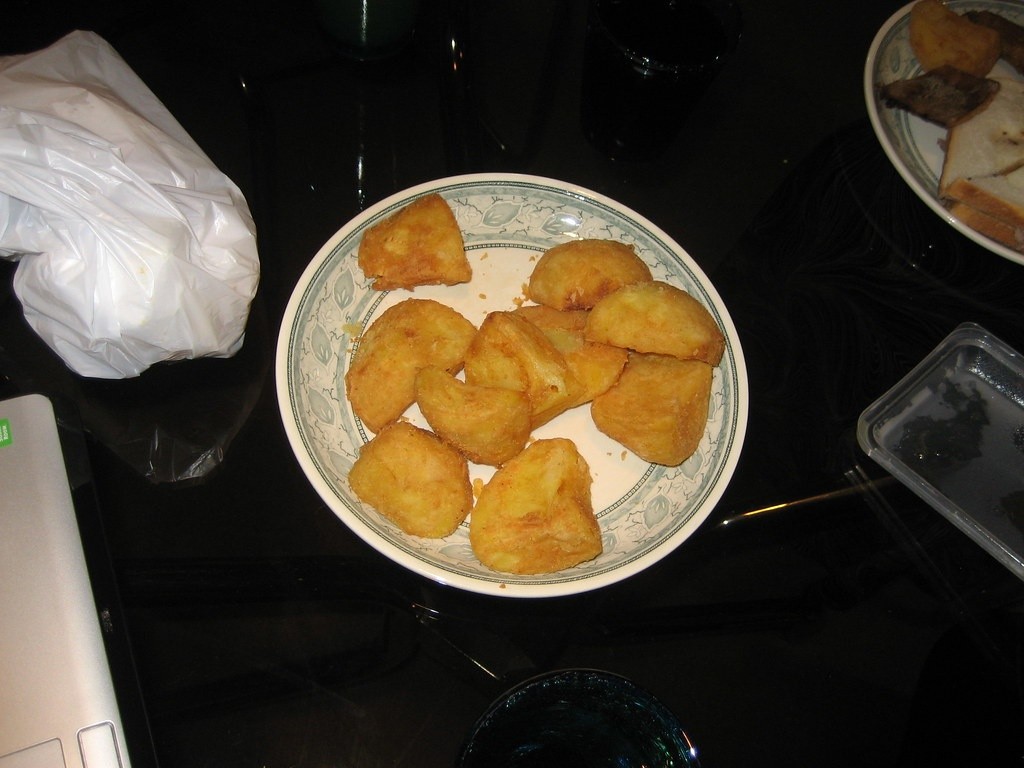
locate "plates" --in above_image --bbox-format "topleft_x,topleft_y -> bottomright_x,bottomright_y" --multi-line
275,173 -> 748,597
460,668 -> 702,768
864,1 -> 1024,264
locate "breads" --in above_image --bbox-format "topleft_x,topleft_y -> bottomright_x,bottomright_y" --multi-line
937,55 -> 1024,256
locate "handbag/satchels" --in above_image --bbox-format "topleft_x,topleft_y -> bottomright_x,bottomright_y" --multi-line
0,30 -> 262,381
